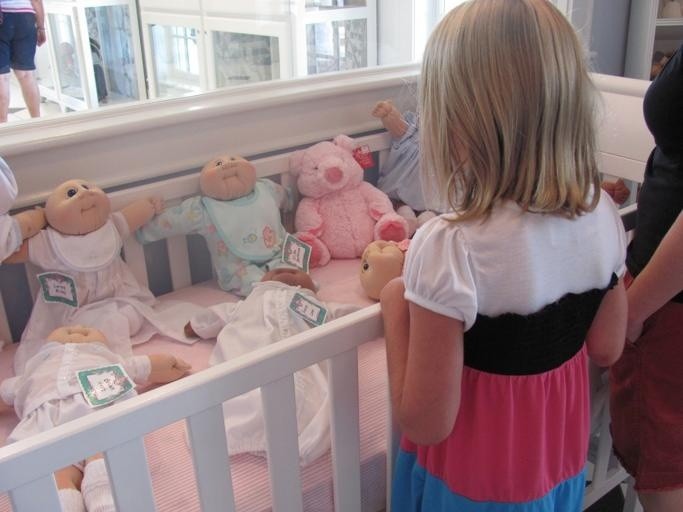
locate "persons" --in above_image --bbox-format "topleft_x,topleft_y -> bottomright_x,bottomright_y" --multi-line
0,2 -> 48,123
360,238 -> 415,301
132,155 -> 298,298
374,100 -> 447,212
1,325 -> 189,510
7,180 -> 163,356
609,48 -> 683,512
383,2 -> 629,512
185,269 -> 368,465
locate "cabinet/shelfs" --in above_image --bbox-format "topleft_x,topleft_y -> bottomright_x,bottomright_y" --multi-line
621,0 -> 683,81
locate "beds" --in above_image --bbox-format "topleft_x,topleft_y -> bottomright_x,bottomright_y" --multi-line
0,128 -> 648,511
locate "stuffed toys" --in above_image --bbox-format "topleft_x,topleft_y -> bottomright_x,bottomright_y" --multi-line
290,136 -> 406,266
